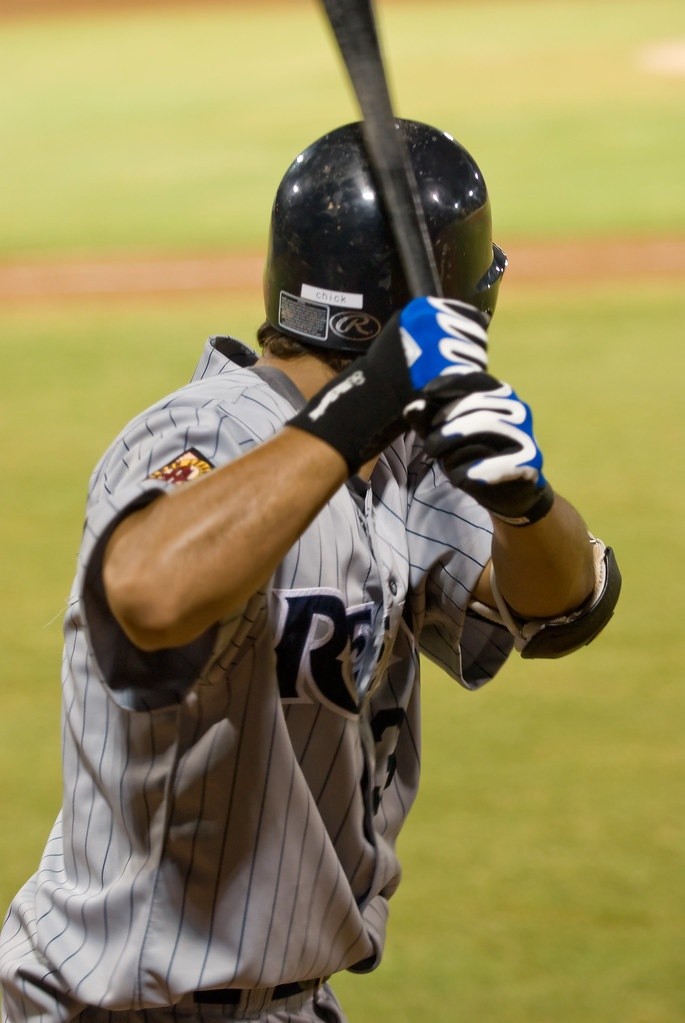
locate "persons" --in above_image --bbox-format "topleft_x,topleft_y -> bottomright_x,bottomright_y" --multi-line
0,115 -> 622,1023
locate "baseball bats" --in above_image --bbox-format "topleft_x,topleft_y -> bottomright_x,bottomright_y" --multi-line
322,0 -> 447,301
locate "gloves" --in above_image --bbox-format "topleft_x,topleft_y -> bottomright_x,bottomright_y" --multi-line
403,373 -> 554,529
283,292 -> 490,484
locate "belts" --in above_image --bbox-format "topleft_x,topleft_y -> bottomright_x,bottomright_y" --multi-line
191,970 -> 336,1009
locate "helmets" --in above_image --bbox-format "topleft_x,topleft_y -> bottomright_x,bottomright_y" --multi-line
261,118 -> 509,354
